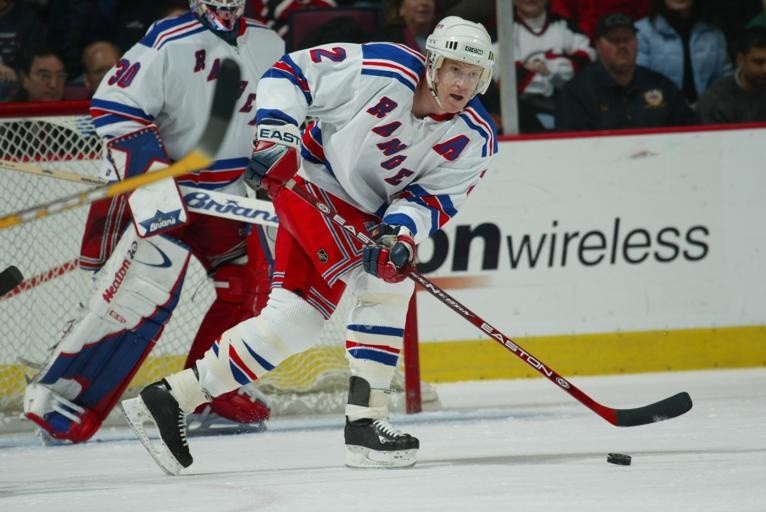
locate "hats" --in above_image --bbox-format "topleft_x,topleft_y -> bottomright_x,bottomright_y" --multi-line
595,11 -> 640,35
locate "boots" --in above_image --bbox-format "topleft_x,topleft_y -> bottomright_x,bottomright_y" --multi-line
137,366 -> 212,470
343,374 -> 422,452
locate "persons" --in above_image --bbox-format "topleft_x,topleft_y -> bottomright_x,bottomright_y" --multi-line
19,0 -> 290,444
135,12 -> 503,468
2,1 -> 766,156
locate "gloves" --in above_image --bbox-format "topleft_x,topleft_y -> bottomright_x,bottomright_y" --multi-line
243,138 -> 301,201
361,220 -> 418,285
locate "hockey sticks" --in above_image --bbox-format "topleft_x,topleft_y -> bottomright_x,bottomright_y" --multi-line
1,59 -> 240,229
285,177 -> 693,426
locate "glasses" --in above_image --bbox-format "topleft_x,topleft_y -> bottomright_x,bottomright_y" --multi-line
28,70 -> 70,81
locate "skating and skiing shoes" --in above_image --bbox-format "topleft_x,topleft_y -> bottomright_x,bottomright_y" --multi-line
198,386 -> 272,424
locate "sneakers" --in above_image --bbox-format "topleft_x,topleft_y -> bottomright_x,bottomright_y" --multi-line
40,408 -> 106,445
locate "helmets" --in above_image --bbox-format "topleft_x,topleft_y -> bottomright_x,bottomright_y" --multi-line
424,15 -> 498,113
188,0 -> 246,33
